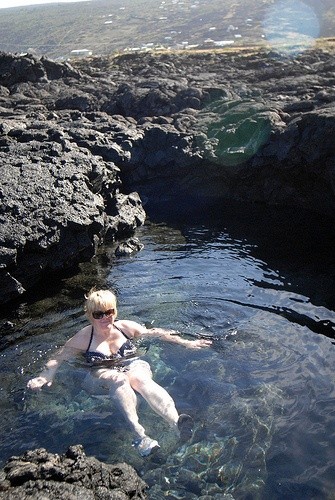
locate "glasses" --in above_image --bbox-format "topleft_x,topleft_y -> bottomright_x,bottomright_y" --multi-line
91,309 -> 114,319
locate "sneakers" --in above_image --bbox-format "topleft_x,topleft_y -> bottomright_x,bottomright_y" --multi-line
172,414 -> 194,444
132,436 -> 160,457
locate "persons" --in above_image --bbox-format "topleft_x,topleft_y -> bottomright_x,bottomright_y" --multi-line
27,288 -> 212,457
